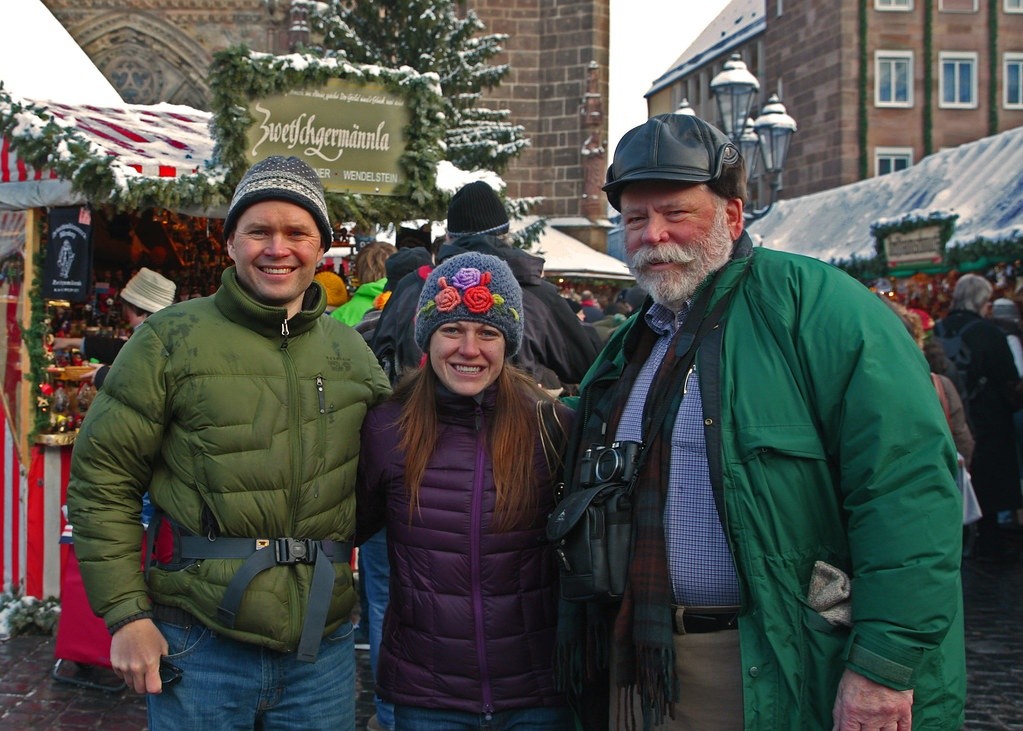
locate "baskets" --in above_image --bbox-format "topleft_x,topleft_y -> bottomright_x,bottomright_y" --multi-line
64,366 -> 96,380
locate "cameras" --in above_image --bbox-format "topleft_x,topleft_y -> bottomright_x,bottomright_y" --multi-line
579,440 -> 642,488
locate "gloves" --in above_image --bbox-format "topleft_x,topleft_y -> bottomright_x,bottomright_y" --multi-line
805,560 -> 854,630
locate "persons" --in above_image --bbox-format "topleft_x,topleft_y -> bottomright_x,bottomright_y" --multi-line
935,273 -> 1023,564
558,283 -> 644,337
357,251 -> 577,731
317,225 -> 435,731
557,114 -> 968,731
372,180 -> 598,396
50,267 -> 176,390
880,295 -> 977,468
67,157 -> 393,731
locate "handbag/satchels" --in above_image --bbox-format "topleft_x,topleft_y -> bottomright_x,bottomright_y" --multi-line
545,479 -> 636,601
930,372 -> 984,526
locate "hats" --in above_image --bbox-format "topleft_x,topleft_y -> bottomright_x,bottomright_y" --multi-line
119,267 -> 176,313
447,181 -> 509,239
383,246 -> 432,293
222,155 -> 334,254
908,308 -> 936,332
313,269 -> 347,306
413,251 -> 524,359
624,285 -> 646,310
991,298 -> 1020,322
614,288 -> 628,303
600,115 -> 749,212
563,297 -> 582,314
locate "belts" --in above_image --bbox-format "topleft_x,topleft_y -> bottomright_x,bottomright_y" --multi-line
673,608 -> 740,635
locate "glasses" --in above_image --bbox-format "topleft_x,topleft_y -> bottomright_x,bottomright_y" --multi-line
159,660 -> 183,686
